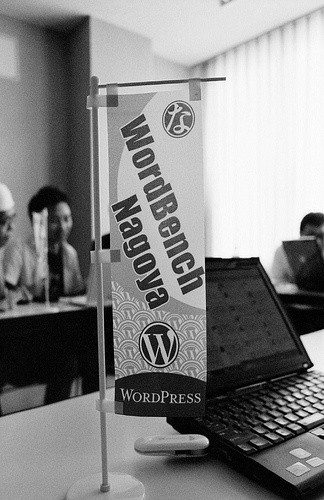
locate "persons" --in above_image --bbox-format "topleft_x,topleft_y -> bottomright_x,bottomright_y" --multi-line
270,213 -> 324,336
0,182 -> 107,406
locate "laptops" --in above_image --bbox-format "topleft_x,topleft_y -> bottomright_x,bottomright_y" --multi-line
282,238 -> 324,293
68,262 -> 111,307
163,256 -> 324,500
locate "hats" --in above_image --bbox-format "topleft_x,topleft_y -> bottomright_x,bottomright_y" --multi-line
0,183 -> 15,212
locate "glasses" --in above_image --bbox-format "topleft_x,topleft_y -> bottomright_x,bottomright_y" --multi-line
0,213 -> 16,225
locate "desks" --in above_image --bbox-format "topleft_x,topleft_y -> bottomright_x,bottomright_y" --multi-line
0,328 -> 324,500
275,283 -> 324,310
0,291 -> 114,391
